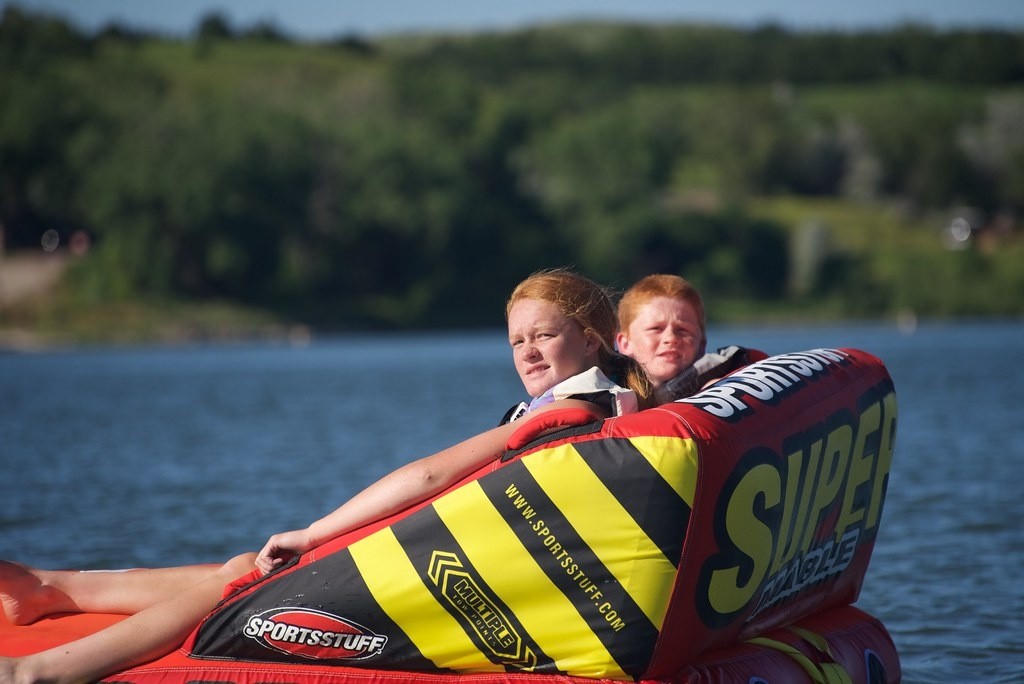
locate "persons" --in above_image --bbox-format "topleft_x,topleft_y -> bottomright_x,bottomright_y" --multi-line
617,274 -> 706,384
0,271 -> 649,684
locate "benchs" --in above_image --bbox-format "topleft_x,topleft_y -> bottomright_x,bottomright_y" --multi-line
185,344 -> 900,684
